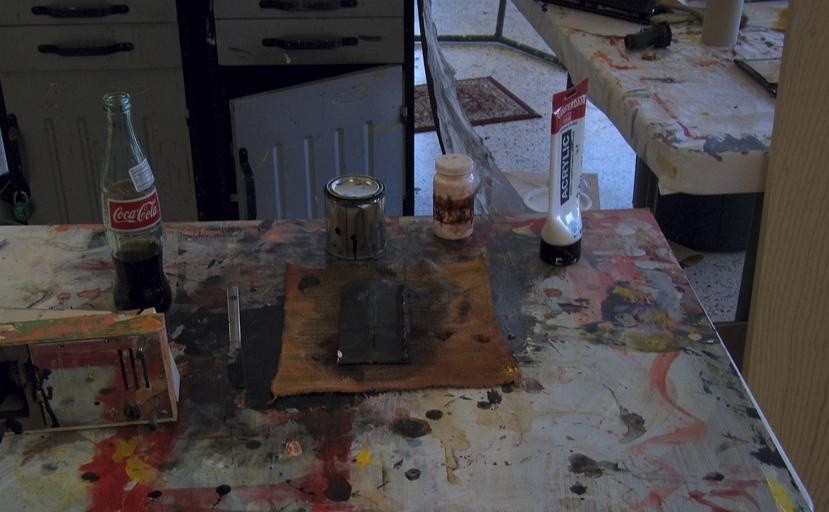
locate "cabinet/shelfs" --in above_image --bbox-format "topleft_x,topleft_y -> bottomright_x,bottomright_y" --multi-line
0,1 -> 414,225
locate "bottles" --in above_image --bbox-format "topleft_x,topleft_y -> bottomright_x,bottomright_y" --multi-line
100,90 -> 173,314
433,152 -> 478,239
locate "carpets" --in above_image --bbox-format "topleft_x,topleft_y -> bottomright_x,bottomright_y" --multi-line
414,75 -> 542,132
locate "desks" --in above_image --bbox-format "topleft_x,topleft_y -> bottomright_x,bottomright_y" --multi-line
0,206 -> 815,512
415,0 -> 789,249
